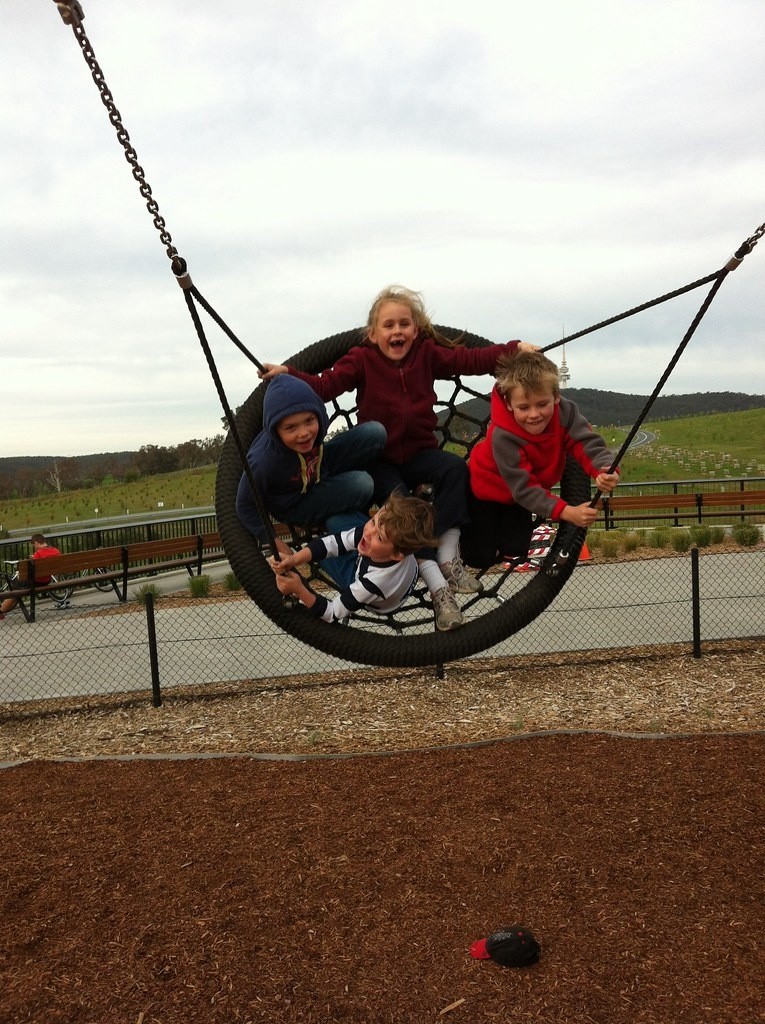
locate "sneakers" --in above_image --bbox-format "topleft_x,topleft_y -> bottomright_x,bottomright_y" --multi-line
436,559 -> 484,594
431,587 -> 464,632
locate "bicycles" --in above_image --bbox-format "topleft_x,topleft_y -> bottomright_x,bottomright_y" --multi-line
0,560 -> 69,608
46,545 -> 117,603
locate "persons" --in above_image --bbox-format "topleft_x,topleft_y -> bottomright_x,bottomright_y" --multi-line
268,489 -> 437,625
459,351 -> 620,569
234,374 -> 388,559
255,287 -> 544,633
0,533 -> 61,620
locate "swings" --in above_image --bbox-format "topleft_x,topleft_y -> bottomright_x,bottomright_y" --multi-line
56,0 -> 765,669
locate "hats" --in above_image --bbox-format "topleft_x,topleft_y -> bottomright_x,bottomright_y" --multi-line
470,926 -> 540,968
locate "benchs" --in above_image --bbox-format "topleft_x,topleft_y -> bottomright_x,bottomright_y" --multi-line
5,522 -> 304,623
591,489 -> 765,531
